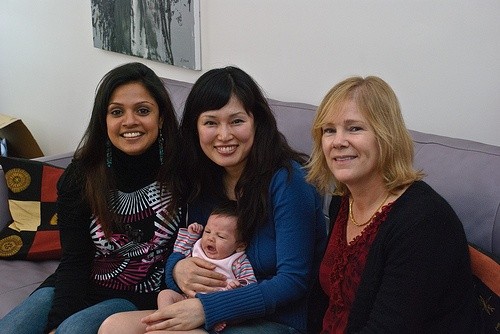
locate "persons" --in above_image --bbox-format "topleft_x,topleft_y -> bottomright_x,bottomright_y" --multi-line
0,62 -> 185,334
146,207 -> 257,330
97,66 -> 327,334
306,76 -> 474,334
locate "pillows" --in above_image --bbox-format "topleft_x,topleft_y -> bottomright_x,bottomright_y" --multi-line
467,242 -> 500,334
0,153 -> 67,261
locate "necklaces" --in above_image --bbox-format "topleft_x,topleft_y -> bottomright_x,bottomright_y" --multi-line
349,193 -> 391,227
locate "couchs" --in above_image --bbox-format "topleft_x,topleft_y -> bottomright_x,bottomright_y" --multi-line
0,77 -> 500,334
